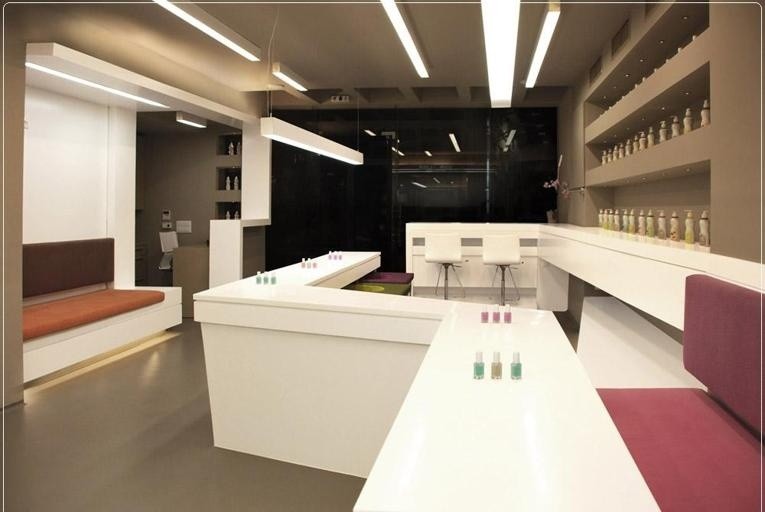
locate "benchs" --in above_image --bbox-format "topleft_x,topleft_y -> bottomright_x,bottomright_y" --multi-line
594,273 -> 761,512
23,237 -> 182,393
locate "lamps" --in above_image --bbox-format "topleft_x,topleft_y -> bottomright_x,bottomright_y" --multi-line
260,6 -> 364,168
525,2 -> 561,87
271,62 -> 309,92
480,1 -> 520,108
156,3 -> 260,62
176,111 -> 207,129
381,3 -> 430,79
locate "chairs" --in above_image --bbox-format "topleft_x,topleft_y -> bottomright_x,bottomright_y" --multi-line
424,232 -> 465,300
482,234 -> 520,305
158,231 -> 179,270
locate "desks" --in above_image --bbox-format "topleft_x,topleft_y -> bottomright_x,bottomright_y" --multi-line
172,241 -> 209,318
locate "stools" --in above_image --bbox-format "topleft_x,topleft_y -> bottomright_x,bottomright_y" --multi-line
360,271 -> 414,283
341,283 -> 412,296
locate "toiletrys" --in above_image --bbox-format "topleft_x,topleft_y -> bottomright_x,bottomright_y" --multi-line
480,304 -> 489,324
256,271 -> 277,284
492,303 -> 501,324
329,251 -> 343,260
473,351 -> 484,379
504,303 -> 512,324
490,351 -> 502,379
511,352 -> 522,380
301,257 -> 317,268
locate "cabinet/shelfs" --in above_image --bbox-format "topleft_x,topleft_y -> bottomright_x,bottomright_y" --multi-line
212,131 -> 242,219
581,3 -> 711,254
